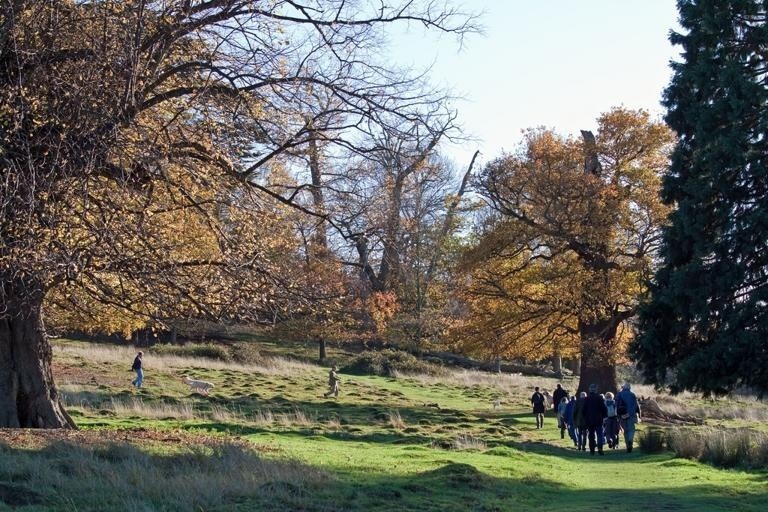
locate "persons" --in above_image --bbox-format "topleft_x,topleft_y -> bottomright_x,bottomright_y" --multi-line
132,350 -> 146,388
530,385 -> 546,430
553,384 -> 641,456
323,365 -> 341,400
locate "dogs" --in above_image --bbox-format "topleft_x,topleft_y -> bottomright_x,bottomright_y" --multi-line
542,391 -> 554,408
181,376 -> 215,395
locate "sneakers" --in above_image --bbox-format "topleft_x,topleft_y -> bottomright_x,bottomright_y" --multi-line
578,448 -> 603,455
607,440 -> 616,449
